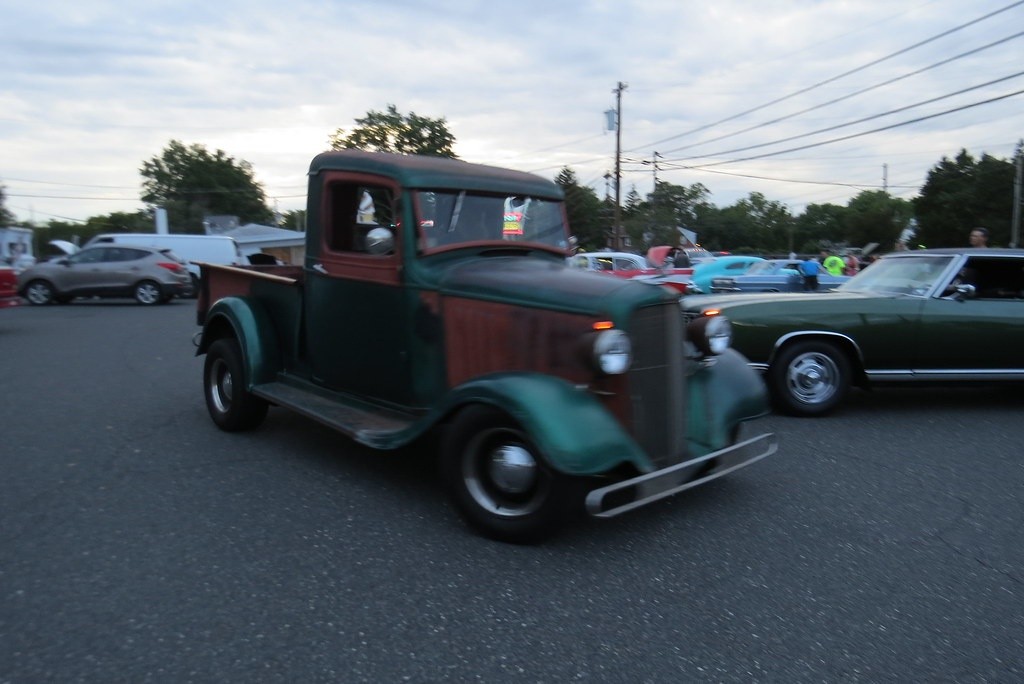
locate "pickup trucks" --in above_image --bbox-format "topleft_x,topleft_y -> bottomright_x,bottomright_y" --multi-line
187,146 -> 781,542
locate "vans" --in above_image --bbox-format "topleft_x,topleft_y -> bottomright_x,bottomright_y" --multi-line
47,233 -> 243,300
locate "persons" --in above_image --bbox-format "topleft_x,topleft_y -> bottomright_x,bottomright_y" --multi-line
968,227 -> 990,248
893,238 -> 911,253
823,250 -> 847,275
844,251 -> 860,277
798,256 -> 823,293
673,248 -> 690,267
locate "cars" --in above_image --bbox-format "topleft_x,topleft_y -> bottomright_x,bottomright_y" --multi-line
676,244 -> 1024,418
709,259 -> 857,295
15,241 -> 187,306
689,255 -> 800,298
646,244 -> 719,275
561,251 -> 665,283
1,261 -> 27,301
